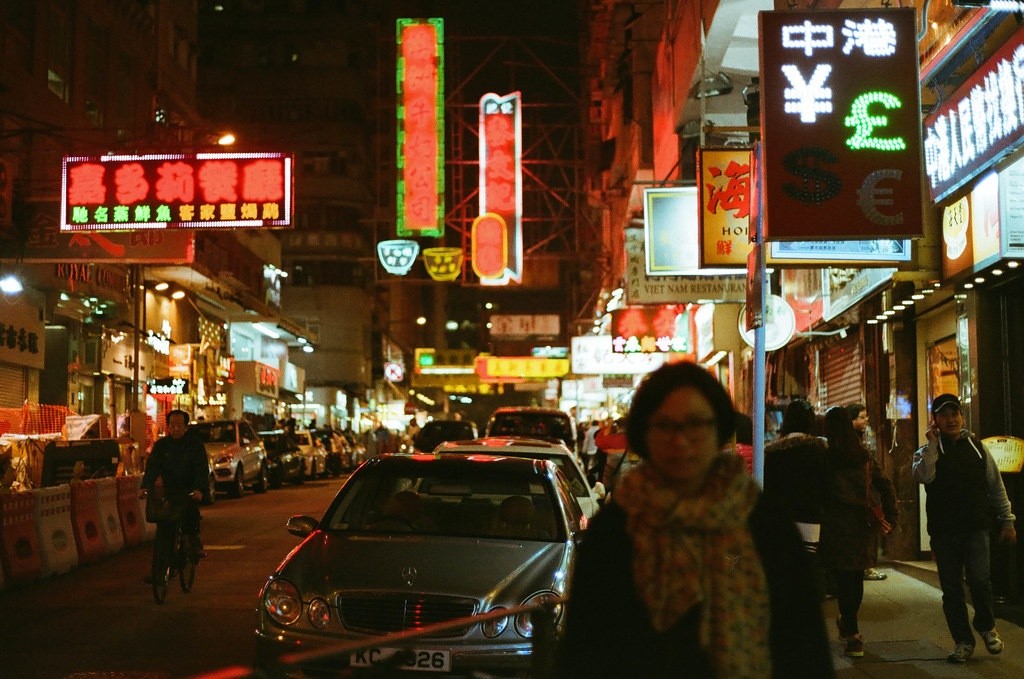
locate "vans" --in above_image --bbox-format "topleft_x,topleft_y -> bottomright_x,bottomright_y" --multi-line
413,420 -> 478,453
485,405 -> 580,458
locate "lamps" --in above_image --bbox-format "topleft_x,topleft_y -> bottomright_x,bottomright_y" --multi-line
863,281 -> 942,327
681,121 -> 700,139
154,281 -> 169,290
303,343 -> 314,353
697,71 -> 733,100
171,284 -> 186,299
297,337 -> 307,344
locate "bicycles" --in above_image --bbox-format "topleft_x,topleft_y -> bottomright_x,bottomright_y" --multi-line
143,491 -> 208,605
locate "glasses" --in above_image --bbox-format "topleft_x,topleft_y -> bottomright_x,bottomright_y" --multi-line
646,413 -> 722,443
856,413 -> 871,425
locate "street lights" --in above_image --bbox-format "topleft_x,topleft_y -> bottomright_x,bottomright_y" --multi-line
741,75 -> 767,497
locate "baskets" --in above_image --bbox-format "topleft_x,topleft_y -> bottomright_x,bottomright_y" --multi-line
146,493 -> 193,524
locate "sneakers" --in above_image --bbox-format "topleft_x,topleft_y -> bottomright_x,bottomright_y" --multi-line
948,641 -> 974,664
979,627 -> 1005,654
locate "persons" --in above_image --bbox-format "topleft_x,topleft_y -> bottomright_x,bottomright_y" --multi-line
913,394 -> 1015,660
139,410 -> 209,583
577,399 -> 895,657
561,360 -> 836,679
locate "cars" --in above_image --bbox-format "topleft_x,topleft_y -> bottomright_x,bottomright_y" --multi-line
315,430 -> 414,477
256,431 -> 303,490
294,429 -> 329,482
411,436 -> 606,520
252,453 -> 601,678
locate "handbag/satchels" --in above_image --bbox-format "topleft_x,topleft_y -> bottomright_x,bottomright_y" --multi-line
865,459 -> 894,536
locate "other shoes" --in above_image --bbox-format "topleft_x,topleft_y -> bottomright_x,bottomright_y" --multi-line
836,614 -> 848,641
844,637 -> 866,657
144,565 -> 166,584
189,533 -> 204,555
862,566 -> 887,580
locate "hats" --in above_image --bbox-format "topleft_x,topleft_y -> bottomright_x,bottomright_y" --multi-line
932,393 -> 961,416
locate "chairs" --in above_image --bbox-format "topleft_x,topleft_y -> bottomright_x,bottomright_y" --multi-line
486,495 -> 551,538
372,491 -> 439,532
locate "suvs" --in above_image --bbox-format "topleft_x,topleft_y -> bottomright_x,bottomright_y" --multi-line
188,418 -> 269,501
